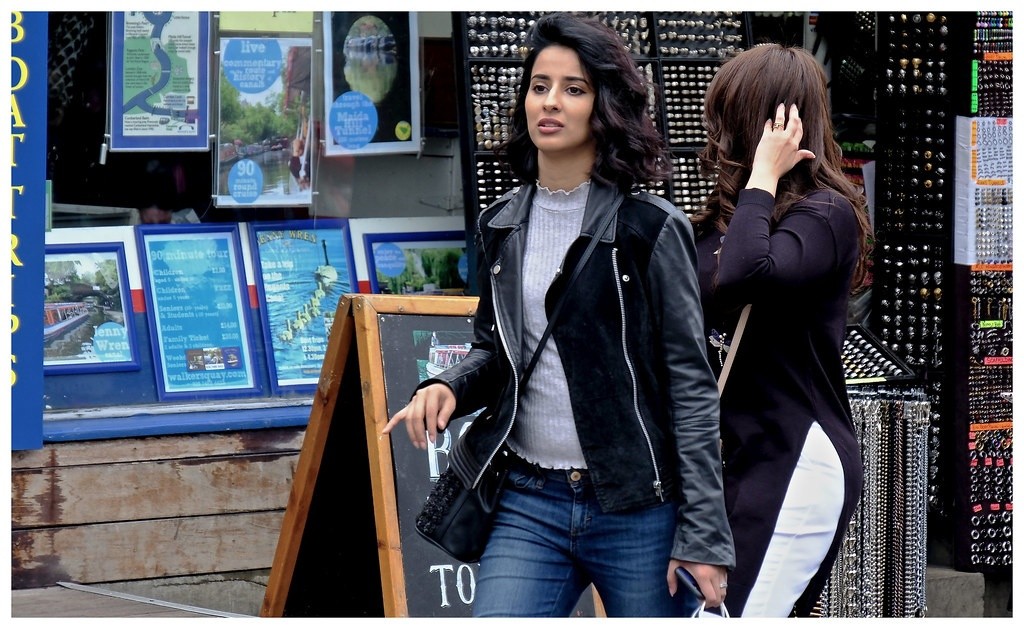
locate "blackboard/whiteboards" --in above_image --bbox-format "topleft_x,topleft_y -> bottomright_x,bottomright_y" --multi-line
259,293 -> 607,618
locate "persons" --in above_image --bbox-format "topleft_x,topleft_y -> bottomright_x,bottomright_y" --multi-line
287,140 -> 304,178
346,24 -> 396,93
382,13 -> 737,617
63,306 -> 85,320
685,43 -> 872,617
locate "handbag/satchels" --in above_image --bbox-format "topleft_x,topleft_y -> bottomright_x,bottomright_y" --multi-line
414,445 -> 514,564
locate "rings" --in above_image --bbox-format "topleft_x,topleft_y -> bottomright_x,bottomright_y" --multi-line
841,330 -> 903,379
467,11 -> 744,218
719,583 -> 727,588
773,123 -> 784,129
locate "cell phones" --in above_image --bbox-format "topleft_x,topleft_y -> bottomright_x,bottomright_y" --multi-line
674,565 -> 706,601
